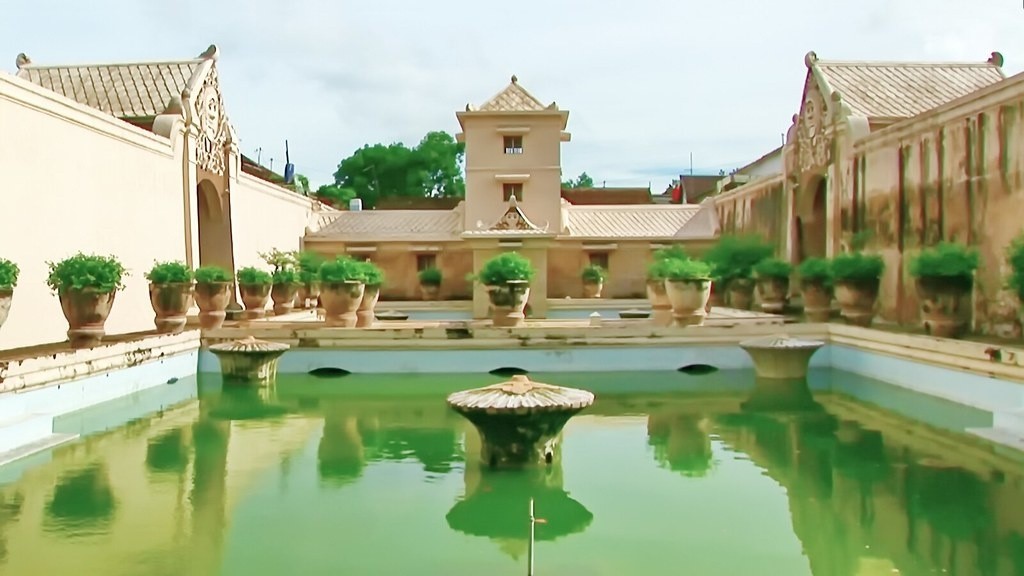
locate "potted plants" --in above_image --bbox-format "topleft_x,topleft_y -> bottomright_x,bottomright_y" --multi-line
478,251 -> 534,325
909,241 -> 982,338
0,257 -> 20,327
583,266 -> 607,298
645,232 -> 885,329
419,266 -> 443,299
45,250 -> 131,348
145,245 -> 387,329
